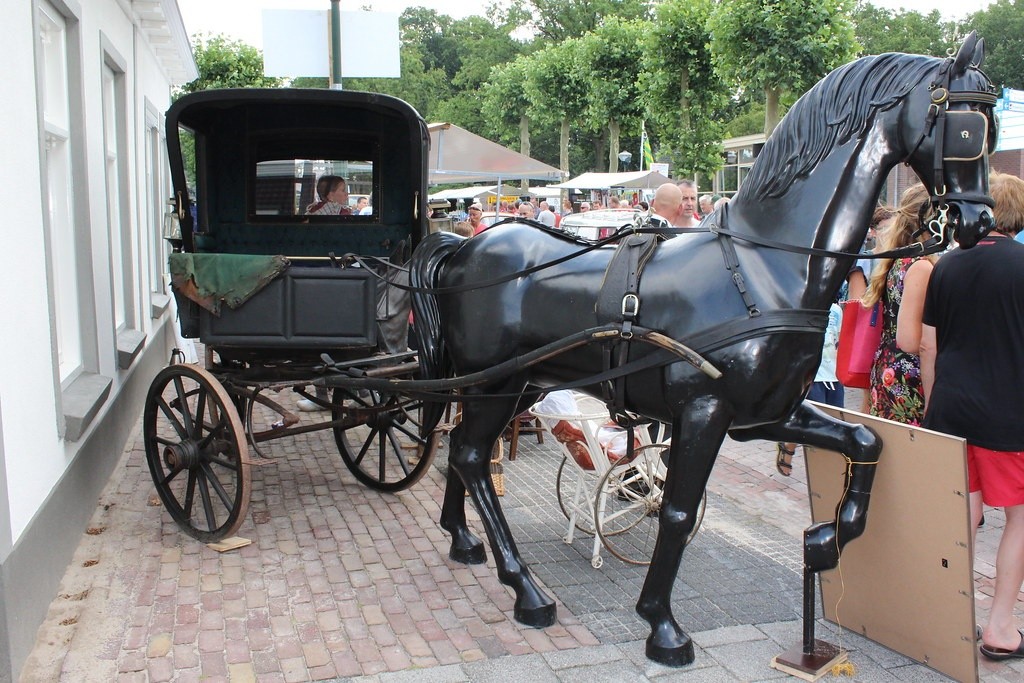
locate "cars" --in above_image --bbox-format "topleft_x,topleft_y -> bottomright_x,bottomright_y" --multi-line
480,211 -> 516,226
143,30 -> 1001,665
560,210 -> 675,244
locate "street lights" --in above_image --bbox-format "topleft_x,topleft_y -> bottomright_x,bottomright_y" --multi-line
618,149 -> 633,171
726,153 -> 737,191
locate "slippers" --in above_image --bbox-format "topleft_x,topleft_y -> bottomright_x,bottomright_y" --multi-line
976,624 -> 983,641
979,630 -> 1024,660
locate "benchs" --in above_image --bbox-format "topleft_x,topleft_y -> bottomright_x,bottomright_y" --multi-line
191,221 -> 413,275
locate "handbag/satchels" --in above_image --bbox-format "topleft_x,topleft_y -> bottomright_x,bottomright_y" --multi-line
836,300 -> 884,389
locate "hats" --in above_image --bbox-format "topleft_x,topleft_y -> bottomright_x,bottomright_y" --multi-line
468,202 -> 482,212
712,195 -> 721,202
581,202 -> 590,208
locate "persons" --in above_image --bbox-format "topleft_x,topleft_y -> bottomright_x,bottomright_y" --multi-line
774,163 -> 1024,660
455,179 -> 732,243
616,415 -> 673,517
304,175 -> 373,215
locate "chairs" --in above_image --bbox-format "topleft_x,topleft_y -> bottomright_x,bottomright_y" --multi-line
528,401 -> 671,569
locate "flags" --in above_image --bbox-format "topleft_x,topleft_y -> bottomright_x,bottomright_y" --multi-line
643,127 -> 654,170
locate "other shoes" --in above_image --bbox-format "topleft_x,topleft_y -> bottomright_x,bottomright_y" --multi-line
297,399 -> 329,412
978,515 -> 984,527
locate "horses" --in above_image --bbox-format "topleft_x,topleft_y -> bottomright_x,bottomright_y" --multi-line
406,28 -> 1001,668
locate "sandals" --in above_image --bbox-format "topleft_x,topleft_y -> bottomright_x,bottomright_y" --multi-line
777,441 -> 795,476
